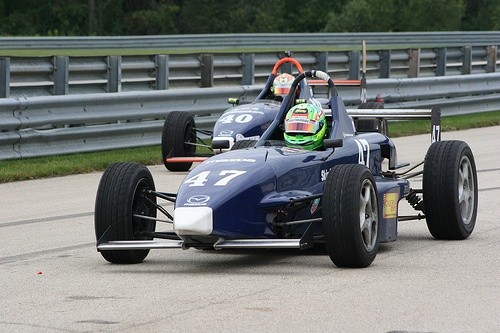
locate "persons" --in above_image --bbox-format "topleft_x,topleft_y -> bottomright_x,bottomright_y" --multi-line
282,102 -> 328,152
270,74 -> 299,102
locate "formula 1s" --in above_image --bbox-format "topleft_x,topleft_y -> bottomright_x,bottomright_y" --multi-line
95,70 -> 479,269
161,58 -> 389,172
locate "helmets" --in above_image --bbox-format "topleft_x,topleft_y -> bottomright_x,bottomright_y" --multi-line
283,102 -> 328,151
273,73 -> 299,98
